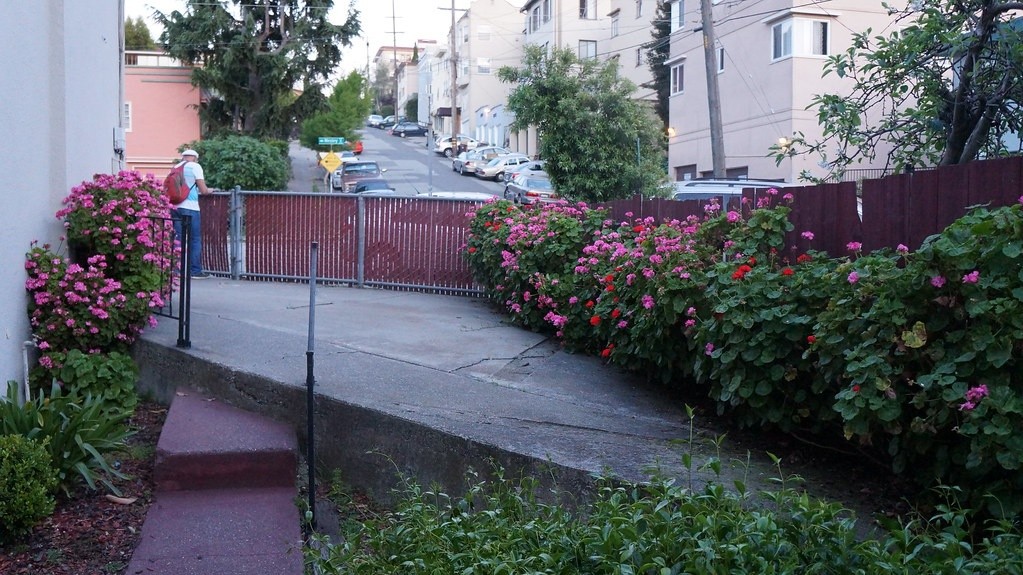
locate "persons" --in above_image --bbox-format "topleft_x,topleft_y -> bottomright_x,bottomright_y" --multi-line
170,150 -> 214,280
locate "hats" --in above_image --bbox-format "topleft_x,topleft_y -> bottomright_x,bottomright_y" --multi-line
182,150 -> 198,158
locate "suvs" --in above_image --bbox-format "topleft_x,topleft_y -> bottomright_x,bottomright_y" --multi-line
657,177 -> 793,201
373,115 -> 409,131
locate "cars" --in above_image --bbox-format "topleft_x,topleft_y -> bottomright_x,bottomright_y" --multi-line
391,122 -> 434,138
433,136 -> 479,158
367,115 -> 384,128
504,174 -> 558,206
472,146 -> 531,165
331,151 -> 395,196
343,137 -> 363,155
474,156 -> 532,182
340,190 -> 526,282
451,152 -> 489,176
503,161 -> 550,187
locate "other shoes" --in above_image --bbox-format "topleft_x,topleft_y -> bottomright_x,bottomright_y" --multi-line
191,272 -> 209,279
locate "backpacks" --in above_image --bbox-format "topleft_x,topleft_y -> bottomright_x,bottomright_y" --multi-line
163,161 -> 196,204
